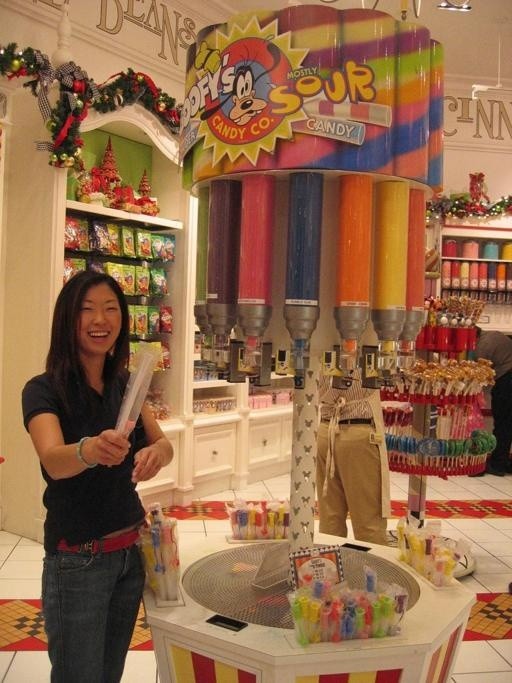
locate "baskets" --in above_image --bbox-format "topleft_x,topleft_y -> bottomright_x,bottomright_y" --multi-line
117,202 -> 142,214
194,396 -> 236,412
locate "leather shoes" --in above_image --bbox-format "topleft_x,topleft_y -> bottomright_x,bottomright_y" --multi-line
484,462 -> 504,476
504,460 -> 512,474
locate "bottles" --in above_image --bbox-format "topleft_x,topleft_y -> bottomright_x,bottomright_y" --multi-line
443,238 -> 457,257
462,239 -> 479,259
500,242 -> 512,260
482,241 -> 499,260
441,260 -> 512,292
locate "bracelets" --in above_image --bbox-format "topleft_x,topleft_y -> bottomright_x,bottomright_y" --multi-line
78,436 -> 98,470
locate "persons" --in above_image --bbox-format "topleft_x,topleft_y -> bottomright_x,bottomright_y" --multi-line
22,271 -> 174,683
316,370 -> 390,546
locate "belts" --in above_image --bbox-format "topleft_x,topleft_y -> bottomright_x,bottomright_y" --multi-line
55,529 -> 140,555
324,417 -> 371,424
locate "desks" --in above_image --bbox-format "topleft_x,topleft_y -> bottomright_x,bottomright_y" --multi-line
144,526 -> 476,682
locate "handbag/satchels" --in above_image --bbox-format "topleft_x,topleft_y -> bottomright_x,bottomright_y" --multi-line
140,502 -> 178,601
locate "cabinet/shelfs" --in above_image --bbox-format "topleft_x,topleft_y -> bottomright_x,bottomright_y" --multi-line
246,372 -> 294,488
0,81 -> 186,545
437,225 -> 512,335
194,324 -> 241,499
